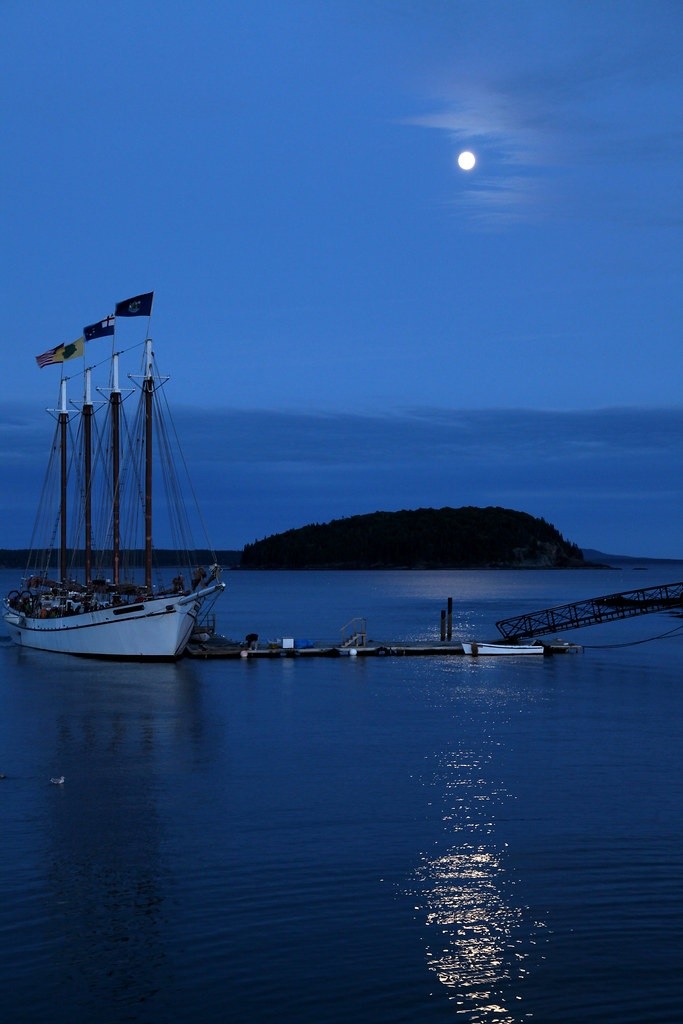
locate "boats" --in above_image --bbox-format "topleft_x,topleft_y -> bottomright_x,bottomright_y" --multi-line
269,650 -> 301,657
323,648 -> 350,657
461,641 -> 544,656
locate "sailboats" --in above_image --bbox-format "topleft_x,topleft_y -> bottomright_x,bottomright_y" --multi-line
1,290 -> 227,661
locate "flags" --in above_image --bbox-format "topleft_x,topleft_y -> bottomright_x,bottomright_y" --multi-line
81,312 -> 114,340
113,291 -> 152,317
54,337 -> 83,360
35,344 -> 63,368
